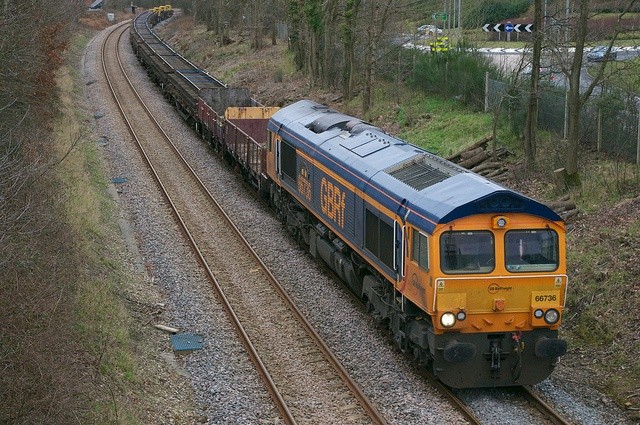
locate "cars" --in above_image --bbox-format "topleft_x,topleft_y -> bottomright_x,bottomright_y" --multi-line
587,45 -> 618,62
418,25 -> 443,34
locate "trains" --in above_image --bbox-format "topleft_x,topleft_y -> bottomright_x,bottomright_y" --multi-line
128,5 -> 568,386
